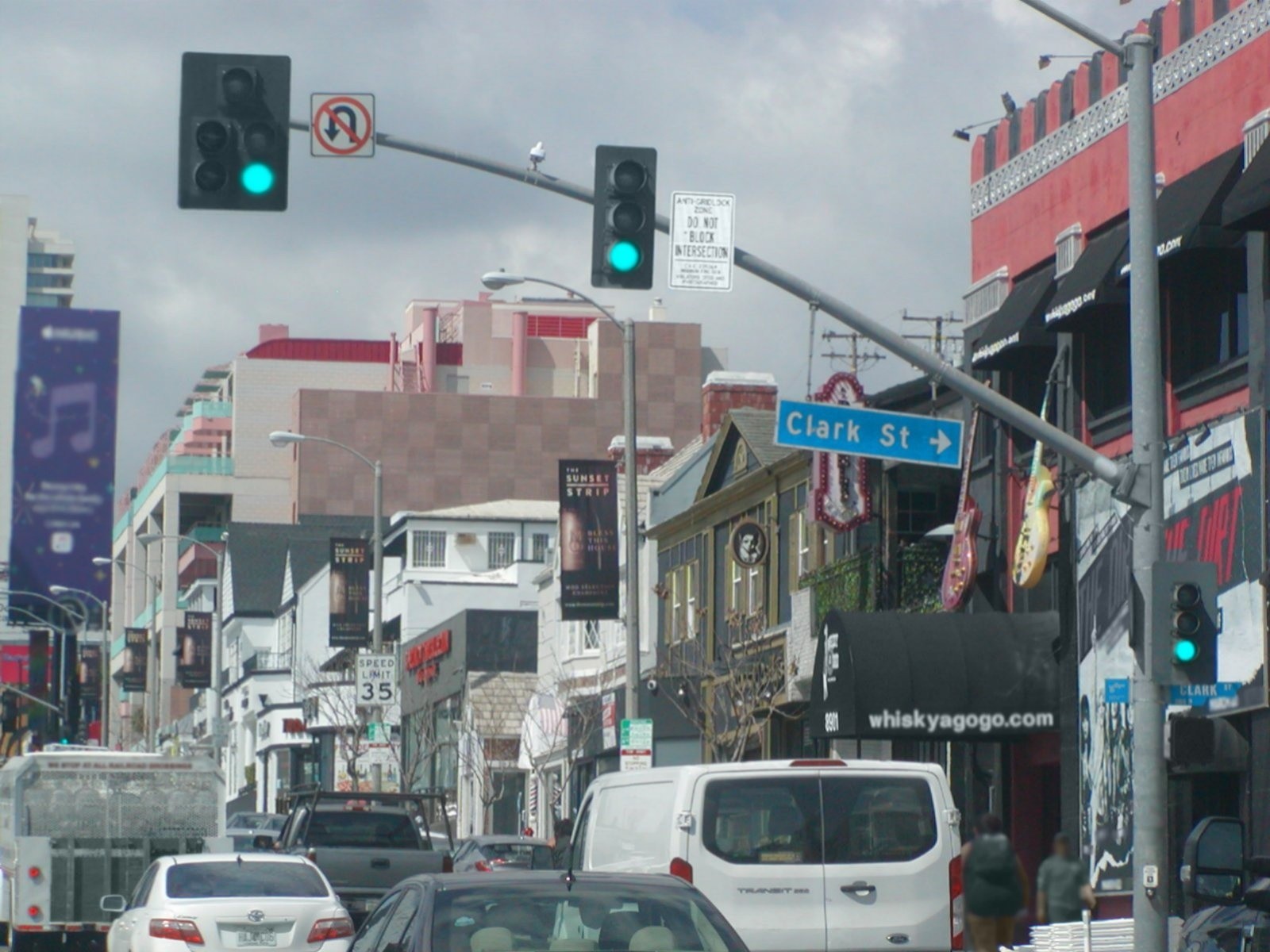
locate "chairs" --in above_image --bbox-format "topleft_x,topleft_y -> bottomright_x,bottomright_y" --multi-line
629,926 -> 679,952
549,938 -> 600,952
469,924 -> 515,952
598,911 -> 649,952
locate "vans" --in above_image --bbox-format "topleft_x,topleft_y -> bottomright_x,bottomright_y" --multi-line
531,756 -> 969,951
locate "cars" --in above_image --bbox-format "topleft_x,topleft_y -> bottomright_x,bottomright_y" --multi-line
98,852 -> 358,952
346,870 -> 747,952
1176,813 -> 1270,952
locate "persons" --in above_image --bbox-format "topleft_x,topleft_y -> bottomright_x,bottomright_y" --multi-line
960,815 -> 1031,952
1035,833 -> 1098,925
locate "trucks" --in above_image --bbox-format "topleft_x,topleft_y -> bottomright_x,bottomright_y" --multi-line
0,747 -> 236,952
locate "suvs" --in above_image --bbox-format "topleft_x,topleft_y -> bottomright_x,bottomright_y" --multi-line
450,834 -> 554,871
225,811 -> 288,853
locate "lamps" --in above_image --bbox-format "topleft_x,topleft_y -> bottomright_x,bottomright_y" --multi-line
1037,54 -> 1093,68
953,117 -> 1003,141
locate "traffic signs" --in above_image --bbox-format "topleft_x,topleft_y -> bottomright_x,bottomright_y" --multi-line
771,396 -> 966,473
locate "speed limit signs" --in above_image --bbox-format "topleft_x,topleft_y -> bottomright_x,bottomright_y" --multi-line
353,654 -> 397,707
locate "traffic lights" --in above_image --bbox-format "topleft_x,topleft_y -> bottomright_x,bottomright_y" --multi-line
590,142 -> 658,291
58,726 -> 74,744
176,50 -> 291,212
1148,558 -> 1218,686
1165,710 -> 1250,778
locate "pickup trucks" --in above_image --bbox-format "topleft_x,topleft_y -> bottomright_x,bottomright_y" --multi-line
249,779 -> 455,931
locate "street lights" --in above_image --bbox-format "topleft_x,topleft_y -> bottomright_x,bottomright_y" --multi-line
478,267 -> 643,772
92,556 -> 158,752
135,531 -> 224,767
48,583 -> 111,747
268,429 -> 385,810
